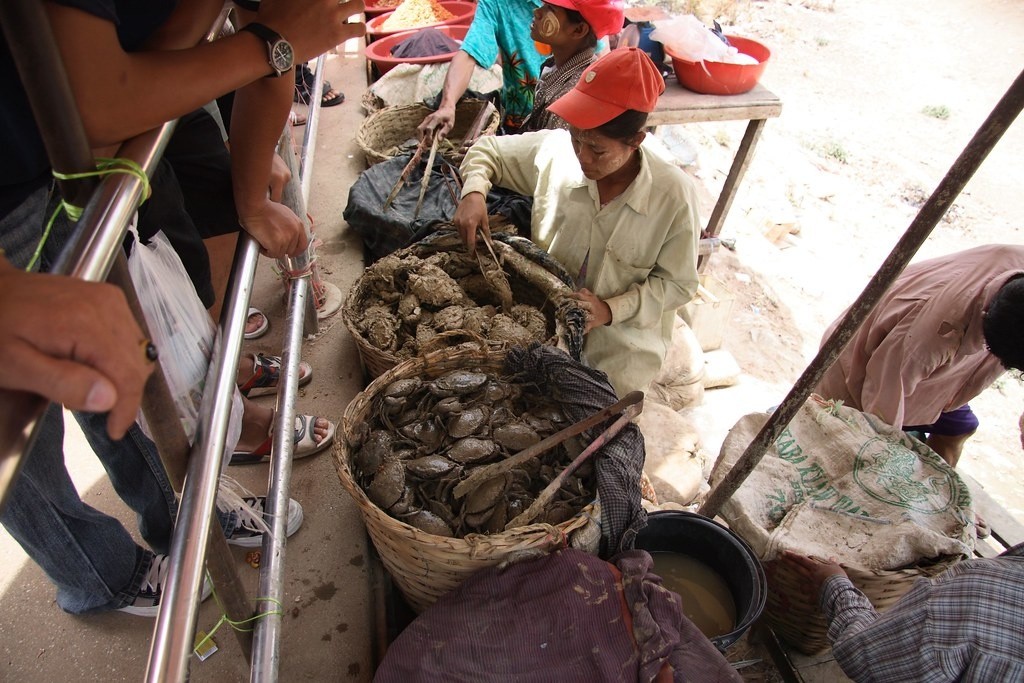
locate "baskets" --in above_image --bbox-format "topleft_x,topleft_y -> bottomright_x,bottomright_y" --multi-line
717,402 -> 976,658
334,96 -> 644,619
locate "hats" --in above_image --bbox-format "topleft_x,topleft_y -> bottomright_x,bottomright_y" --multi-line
545,46 -> 665,130
542,0 -> 626,40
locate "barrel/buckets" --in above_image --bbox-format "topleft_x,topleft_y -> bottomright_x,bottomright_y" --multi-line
633,509 -> 768,656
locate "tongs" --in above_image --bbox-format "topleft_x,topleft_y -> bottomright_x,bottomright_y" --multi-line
384,128 -> 441,220
440,161 -> 501,280
453,390 -> 645,530
458,99 -> 495,148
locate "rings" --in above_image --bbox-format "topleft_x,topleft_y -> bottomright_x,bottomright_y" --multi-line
139,338 -> 158,365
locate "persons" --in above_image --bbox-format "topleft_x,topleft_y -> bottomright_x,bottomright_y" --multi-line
416,0 -> 616,152
775,543 -> 1024,683
465,42 -> 467,43
453,47 -> 700,506
813,244 -> 1024,539
521,0 -> 627,136
0,0 -> 367,618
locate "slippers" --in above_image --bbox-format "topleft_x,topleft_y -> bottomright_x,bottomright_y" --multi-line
245,300 -> 270,339
221,355 -> 338,467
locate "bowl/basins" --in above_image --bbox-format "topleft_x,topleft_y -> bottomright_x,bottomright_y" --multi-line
662,33 -> 771,96
362,0 -> 477,75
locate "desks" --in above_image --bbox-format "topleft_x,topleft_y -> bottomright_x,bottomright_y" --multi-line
636,78 -> 781,279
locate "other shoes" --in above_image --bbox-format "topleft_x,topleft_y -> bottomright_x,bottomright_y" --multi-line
289,113 -> 307,126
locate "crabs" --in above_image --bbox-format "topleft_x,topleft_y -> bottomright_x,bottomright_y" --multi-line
349,248 -> 595,537
395,138 -> 461,156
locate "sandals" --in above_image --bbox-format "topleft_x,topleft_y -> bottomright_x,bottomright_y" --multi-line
293,80 -> 344,108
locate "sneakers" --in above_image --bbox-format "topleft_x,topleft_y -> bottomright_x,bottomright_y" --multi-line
124,494 -> 302,617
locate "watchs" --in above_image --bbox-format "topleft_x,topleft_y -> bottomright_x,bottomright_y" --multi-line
239,21 -> 295,79
